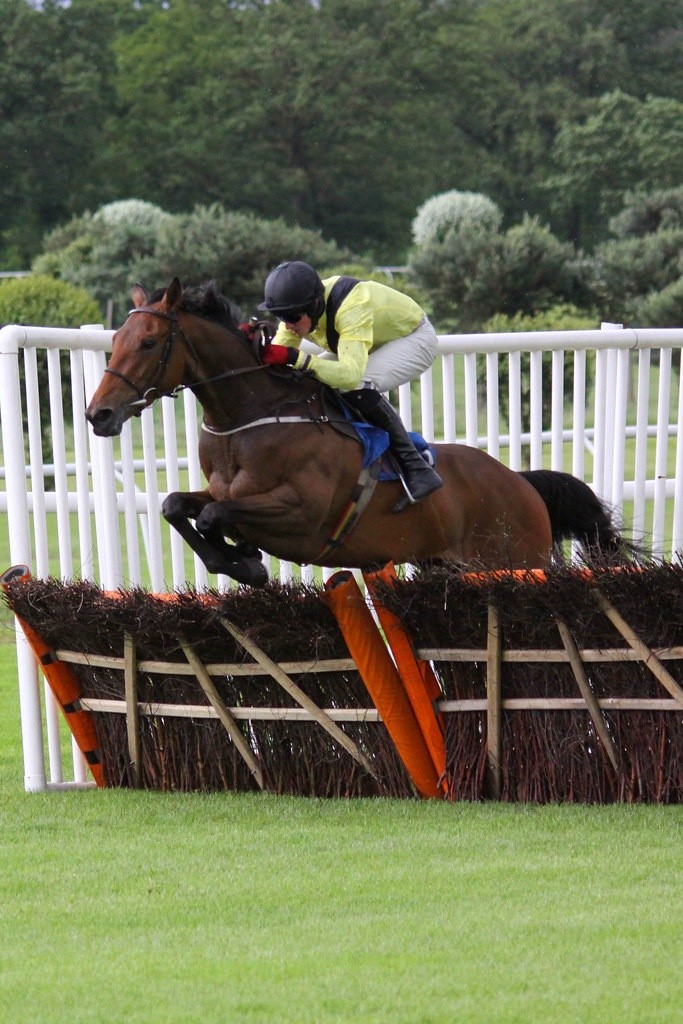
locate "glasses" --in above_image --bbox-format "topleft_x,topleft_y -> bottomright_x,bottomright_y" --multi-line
279,310 -> 305,323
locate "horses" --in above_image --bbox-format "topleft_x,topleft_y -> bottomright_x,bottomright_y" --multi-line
84,274 -> 626,592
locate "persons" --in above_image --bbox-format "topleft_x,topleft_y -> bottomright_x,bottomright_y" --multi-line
263,261 -> 443,515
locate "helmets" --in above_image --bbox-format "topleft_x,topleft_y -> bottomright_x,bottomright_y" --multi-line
257,260 -> 325,311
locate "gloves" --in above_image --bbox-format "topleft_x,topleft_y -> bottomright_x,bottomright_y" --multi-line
263,345 -> 299,365
240,323 -> 261,342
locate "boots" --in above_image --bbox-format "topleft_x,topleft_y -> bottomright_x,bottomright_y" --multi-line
341,387 -> 443,514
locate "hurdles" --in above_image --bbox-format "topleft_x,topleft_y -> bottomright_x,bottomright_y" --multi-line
3,563 -> 682,801
